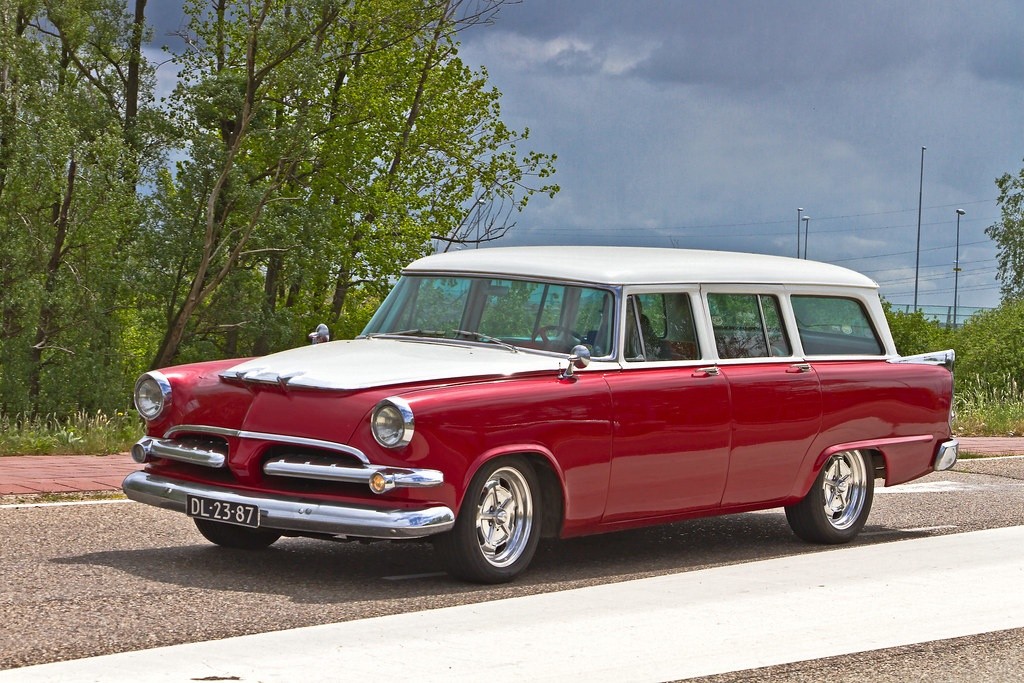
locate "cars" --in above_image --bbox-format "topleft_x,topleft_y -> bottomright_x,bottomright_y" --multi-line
120,242 -> 960,588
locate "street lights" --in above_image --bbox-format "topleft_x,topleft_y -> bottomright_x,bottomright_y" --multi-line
953,207 -> 967,329
475,199 -> 485,249
797,208 -> 803,259
913,145 -> 927,313
802,214 -> 811,260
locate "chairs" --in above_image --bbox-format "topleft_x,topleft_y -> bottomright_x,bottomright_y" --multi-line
640,314 -> 670,359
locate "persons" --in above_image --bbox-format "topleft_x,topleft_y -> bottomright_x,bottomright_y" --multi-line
307,323 -> 333,344
591,294 -> 655,360
